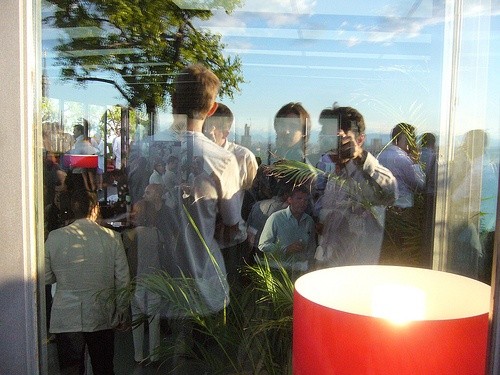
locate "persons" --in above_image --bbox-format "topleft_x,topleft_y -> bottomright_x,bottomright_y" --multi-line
44,189 -> 130,375
122,200 -> 167,368
44,63 -> 498,351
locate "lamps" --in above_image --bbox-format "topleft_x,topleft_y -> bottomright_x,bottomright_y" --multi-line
294,264 -> 493,375
